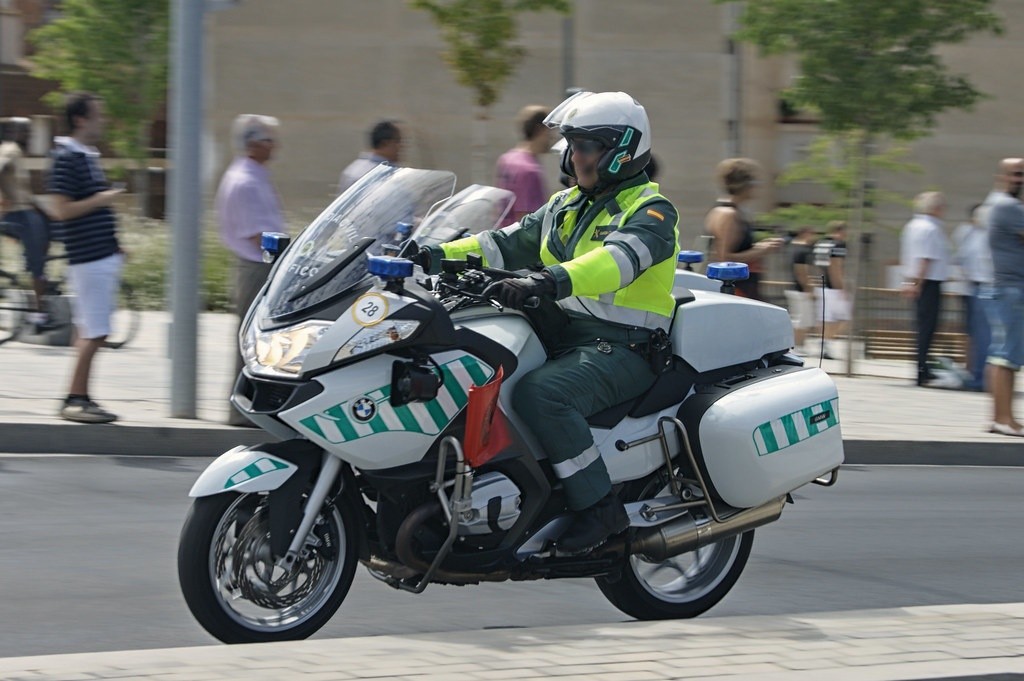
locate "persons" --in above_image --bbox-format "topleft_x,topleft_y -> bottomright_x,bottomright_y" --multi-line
407,91 -> 681,555
814,219 -> 852,360
48,91 -> 129,422
493,104 -> 553,230
0,117 -> 62,296
899,192 -> 948,386
781,227 -> 821,357
216,113 -> 288,428
704,159 -> 783,302
335,120 -> 402,198
958,155 -> 1024,436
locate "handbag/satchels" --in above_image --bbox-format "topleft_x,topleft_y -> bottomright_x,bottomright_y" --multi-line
923,360 -> 974,391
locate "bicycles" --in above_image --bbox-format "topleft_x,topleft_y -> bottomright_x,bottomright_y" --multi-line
0,217 -> 138,349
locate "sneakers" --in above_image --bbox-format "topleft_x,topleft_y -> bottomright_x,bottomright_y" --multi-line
63,394 -> 117,424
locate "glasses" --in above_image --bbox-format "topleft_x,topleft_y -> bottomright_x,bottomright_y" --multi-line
569,138 -> 606,154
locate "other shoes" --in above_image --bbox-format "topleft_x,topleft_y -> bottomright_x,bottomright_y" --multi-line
823,353 -> 832,359
794,346 -> 811,356
38,279 -> 62,296
990,419 -> 1024,437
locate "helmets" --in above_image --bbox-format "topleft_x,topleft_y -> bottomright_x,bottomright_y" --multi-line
542,91 -> 652,188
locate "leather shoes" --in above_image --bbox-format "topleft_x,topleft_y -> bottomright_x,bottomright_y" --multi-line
557,493 -> 630,552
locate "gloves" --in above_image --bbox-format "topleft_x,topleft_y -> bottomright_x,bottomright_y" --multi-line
483,273 -> 557,310
402,246 -> 431,274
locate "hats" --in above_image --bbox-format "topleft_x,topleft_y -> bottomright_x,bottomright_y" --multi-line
236,114 -> 278,142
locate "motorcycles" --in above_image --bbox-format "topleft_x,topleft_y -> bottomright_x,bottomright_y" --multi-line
177,160 -> 845,644
